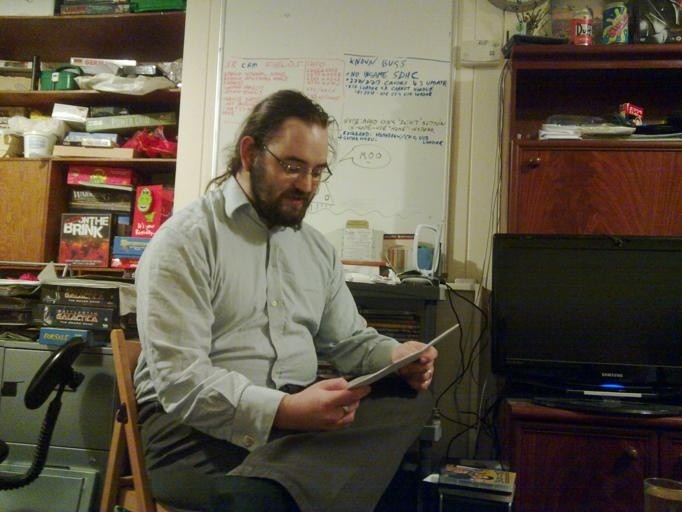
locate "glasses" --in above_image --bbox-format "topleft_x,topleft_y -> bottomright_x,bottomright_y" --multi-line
262,145 -> 333,182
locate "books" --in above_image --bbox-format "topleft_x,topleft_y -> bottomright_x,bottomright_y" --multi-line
439,461 -> 516,502
38,155 -> 167,345
539,120 -> 680,141
347,322 -> 459,391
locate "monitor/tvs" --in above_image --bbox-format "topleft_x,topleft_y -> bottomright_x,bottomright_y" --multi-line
490,233 -> 682,417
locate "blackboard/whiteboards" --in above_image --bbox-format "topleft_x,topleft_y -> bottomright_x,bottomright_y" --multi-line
209,0 -> 459,280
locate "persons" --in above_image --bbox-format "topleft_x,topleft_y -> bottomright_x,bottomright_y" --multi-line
131,92 -> 437,510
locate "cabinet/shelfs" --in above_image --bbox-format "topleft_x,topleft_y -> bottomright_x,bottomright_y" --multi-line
504,396 -> 682,511
1,3 -> 188,278
495,37 -> 681,235
1,336 -> 135,511
314,279 -> 440,398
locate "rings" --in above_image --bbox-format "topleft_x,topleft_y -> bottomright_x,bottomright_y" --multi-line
343,404 -> 351,415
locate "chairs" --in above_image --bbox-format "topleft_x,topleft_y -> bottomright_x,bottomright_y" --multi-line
98,330 -> 176,511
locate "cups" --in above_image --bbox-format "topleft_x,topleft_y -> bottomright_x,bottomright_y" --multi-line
22,132 -> 57,159
